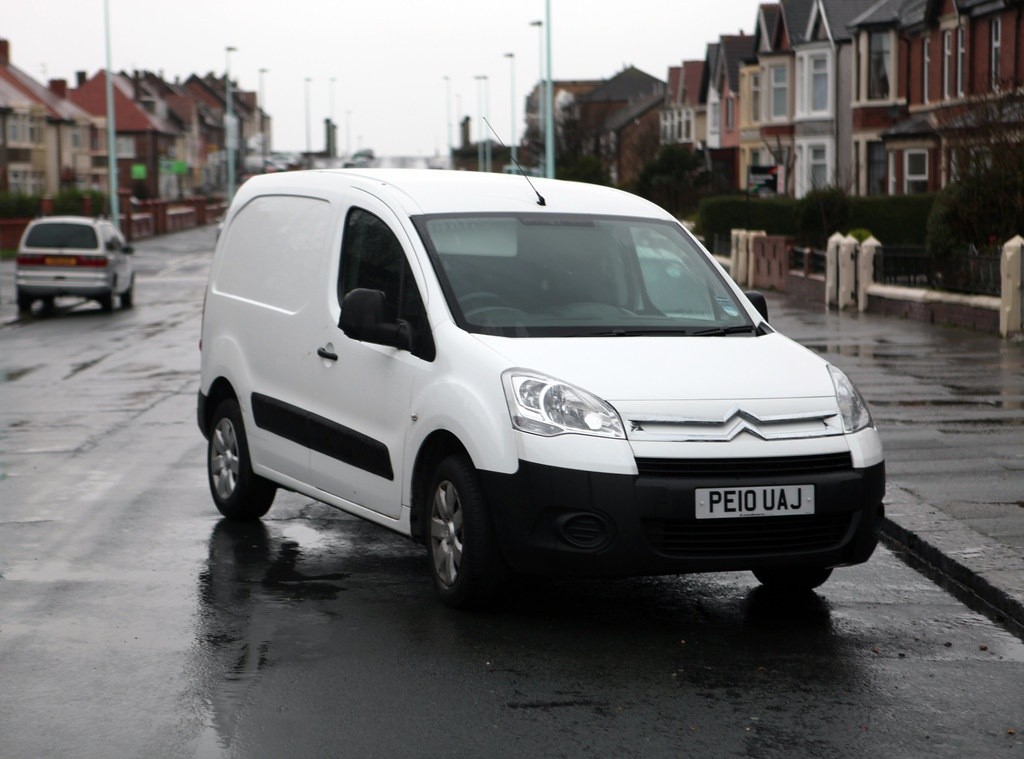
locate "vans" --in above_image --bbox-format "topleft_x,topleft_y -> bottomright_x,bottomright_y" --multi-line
195,163 -> 890,606
14,215 -> 136,314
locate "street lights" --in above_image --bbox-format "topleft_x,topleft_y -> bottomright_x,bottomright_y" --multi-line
225,46 -> 239,201
506,52 -> 519,172
476,74 -> 490,171
531,19 -> 545,177
302,76 -> 313,170
254,68 -> 267,171
